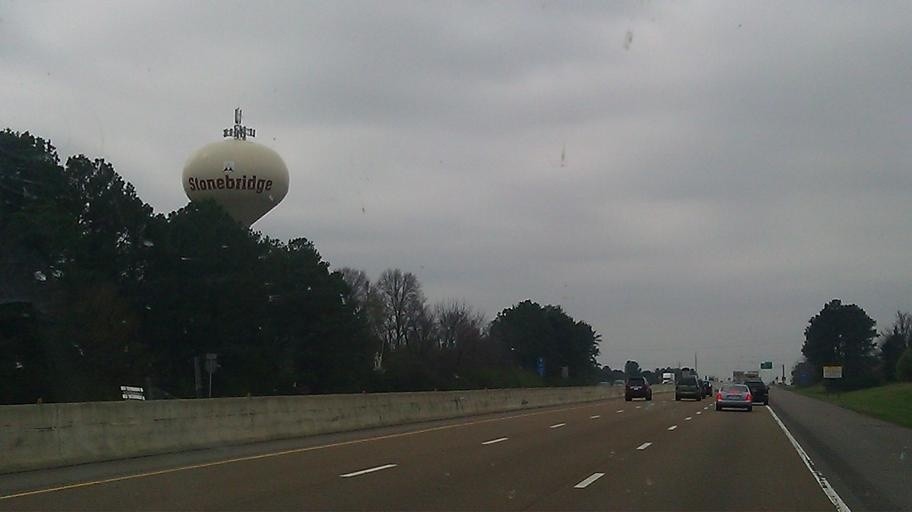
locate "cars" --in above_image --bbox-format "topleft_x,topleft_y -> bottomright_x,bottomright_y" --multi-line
676,375 -> 769,411
625,376 -> 652,401
600,380 -> 625,387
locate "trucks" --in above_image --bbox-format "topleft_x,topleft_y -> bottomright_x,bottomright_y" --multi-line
662,373 -> 675,384
